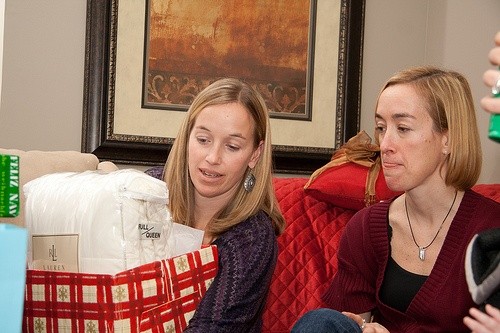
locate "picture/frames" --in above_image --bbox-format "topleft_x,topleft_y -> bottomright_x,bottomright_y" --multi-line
77,0 -> 368,181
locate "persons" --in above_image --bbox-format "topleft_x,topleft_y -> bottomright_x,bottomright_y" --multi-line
139,77 -> 286,333
290,66 -> 500,333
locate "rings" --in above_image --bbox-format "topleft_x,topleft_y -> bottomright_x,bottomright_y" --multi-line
361,319 -> 366,328
491,79 -> 500,96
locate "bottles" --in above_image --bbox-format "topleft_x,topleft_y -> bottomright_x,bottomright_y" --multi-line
488,64 -> 500,141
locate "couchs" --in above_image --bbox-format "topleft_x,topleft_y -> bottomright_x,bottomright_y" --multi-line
0,147 -> 119,237
260,176 -> 500,332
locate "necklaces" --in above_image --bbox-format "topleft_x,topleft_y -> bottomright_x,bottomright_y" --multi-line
404,188 -> 458,260
